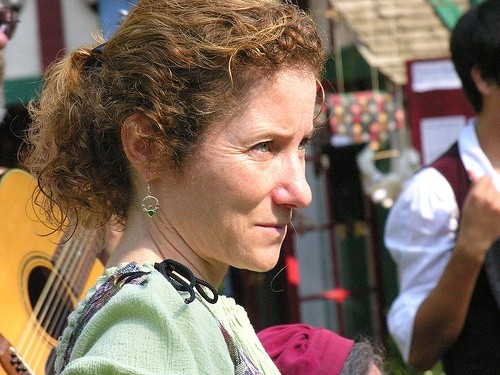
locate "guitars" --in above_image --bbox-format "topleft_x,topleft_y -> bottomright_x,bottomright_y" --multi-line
0,168 -> 105,375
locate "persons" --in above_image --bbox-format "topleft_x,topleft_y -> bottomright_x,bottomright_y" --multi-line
379,1 -> 499,375
15,0 -> 336,375
254,323 -> 389,375
